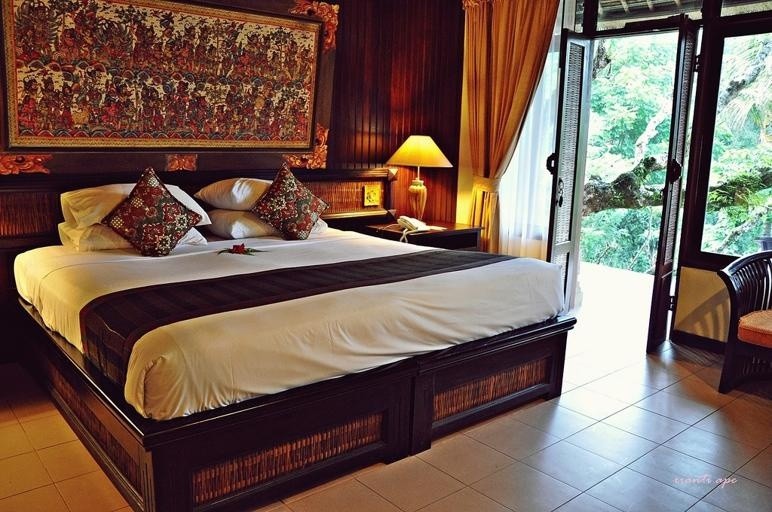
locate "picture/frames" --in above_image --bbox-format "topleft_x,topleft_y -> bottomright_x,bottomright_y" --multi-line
0,0 -> 339,179
363,185 -> 381,206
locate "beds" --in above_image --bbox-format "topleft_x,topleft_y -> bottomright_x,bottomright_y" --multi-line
2,168 -> 577,512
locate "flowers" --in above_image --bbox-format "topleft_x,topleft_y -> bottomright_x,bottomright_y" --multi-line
217,242 -> 269,256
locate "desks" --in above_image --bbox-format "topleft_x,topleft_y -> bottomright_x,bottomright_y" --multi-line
364,221 -> 485,252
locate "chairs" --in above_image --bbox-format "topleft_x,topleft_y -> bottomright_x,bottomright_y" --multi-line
716,249 -> 772,393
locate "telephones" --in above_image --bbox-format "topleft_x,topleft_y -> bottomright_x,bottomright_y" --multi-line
396,216 -> 430,232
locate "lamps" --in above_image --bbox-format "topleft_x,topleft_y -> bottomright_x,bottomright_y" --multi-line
385,135 -> 454,219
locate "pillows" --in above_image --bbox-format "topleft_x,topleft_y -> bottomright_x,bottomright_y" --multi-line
204,208 -> 329,239
100,166 -> 203,257
59,184 -> 212,227
193,177 -> 273,210
57,220 -> 208,252
251,161 -> 329,240
